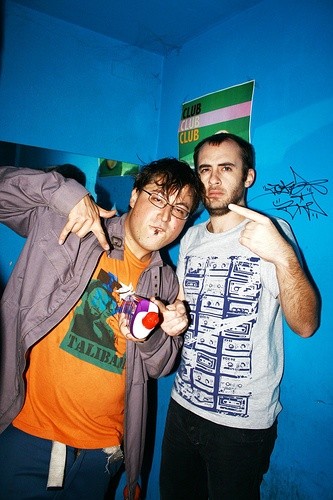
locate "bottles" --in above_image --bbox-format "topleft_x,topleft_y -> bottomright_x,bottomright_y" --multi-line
117,293 -> 159,340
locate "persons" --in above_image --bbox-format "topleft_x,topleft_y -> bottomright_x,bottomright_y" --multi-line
150,133 -> 322,499
1,124 -> 211,500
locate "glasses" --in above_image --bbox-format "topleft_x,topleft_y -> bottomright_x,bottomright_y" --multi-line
140,187 -> 191,220
